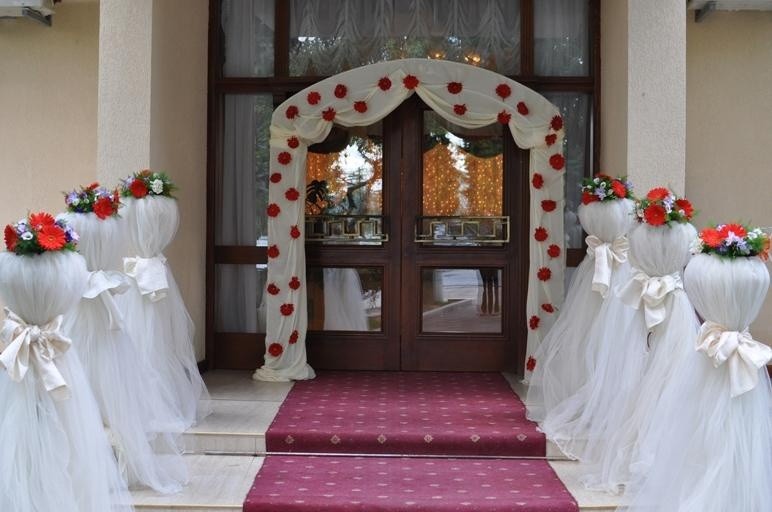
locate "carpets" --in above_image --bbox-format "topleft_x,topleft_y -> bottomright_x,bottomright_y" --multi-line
243,371 -> 581,512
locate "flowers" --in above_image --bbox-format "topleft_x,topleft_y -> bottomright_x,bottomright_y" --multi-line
4,168 -> 180,258
576,172 -> 772,264
